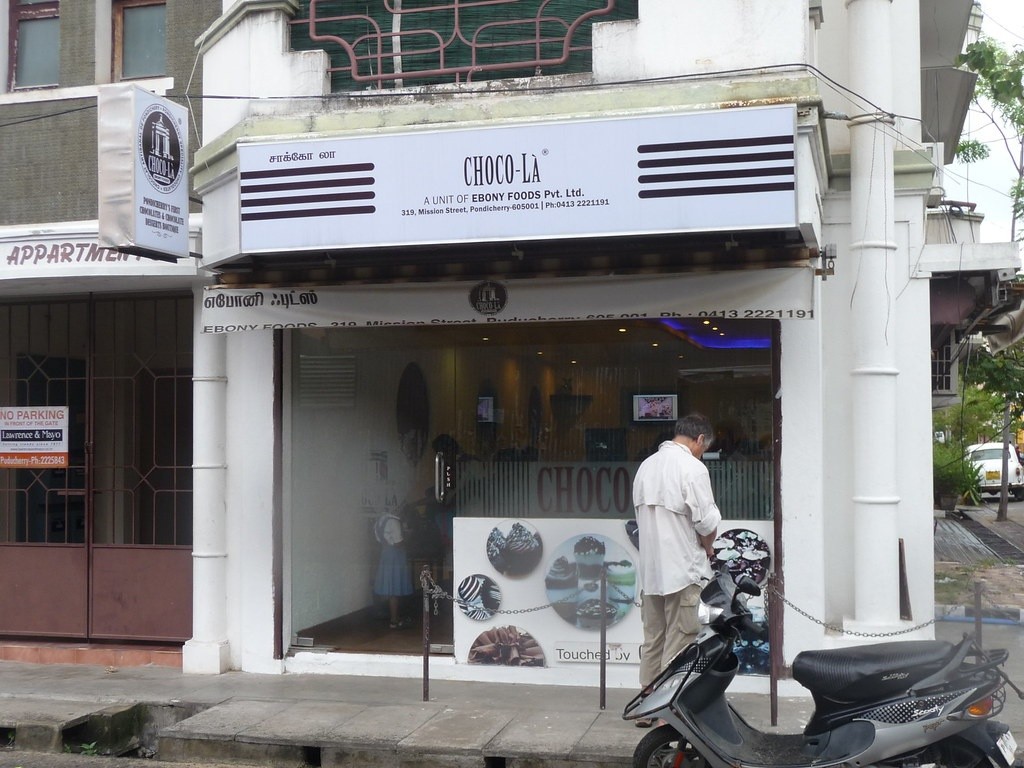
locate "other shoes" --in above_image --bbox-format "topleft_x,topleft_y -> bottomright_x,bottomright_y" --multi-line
390,619 -> 406,629
634,717 -> 657,727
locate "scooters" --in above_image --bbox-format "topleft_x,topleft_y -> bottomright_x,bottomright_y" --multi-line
622,556 -> 1023,768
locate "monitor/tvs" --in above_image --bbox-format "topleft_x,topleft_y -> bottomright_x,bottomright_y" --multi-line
478,397 -> 493,423
633,394 -> 678,421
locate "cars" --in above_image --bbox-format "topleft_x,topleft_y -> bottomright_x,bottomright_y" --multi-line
961,442 -> 1024,501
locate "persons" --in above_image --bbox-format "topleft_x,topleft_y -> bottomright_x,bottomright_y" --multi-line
633,413 -> 722,727
